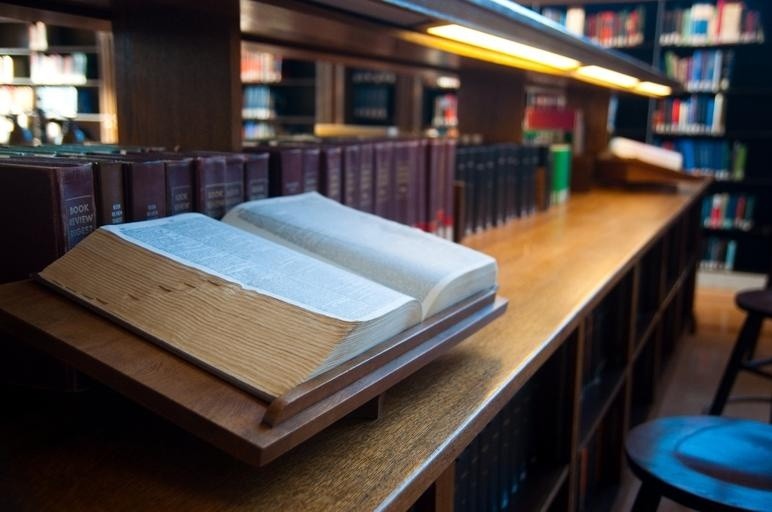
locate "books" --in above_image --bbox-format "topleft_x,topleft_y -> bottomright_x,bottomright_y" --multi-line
31,177 -> 502,414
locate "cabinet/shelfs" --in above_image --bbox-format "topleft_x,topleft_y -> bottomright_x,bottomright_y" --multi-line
644,1 -> 763,275
1,0 -> 713,511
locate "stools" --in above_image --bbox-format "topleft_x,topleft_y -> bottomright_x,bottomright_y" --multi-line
621,288 -> 771,511
702,290 -> 771,419
